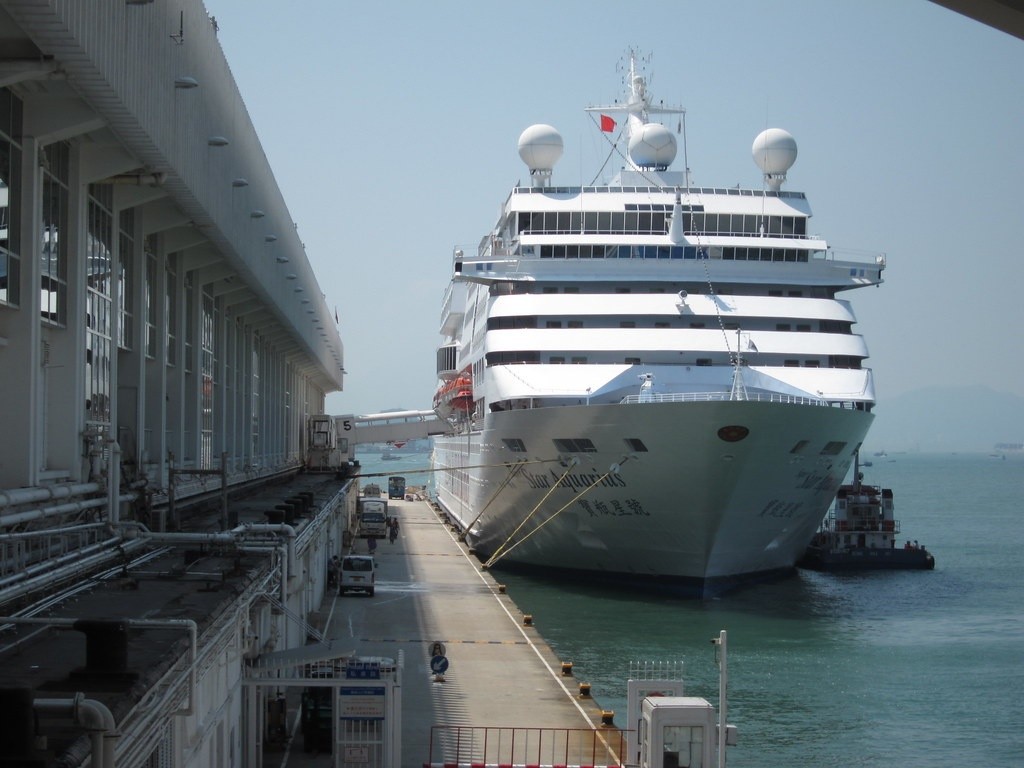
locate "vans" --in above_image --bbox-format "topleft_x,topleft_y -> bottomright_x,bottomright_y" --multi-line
339,556 -> 379,598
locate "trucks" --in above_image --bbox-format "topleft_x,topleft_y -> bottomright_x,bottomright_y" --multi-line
356,501 -> 389,539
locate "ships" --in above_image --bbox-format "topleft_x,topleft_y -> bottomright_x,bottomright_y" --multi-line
426,43 -> 887,596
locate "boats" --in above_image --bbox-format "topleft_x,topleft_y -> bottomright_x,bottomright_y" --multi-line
797,452 -> 936,573
380,448 -> 401,461
431,377 -> 473,424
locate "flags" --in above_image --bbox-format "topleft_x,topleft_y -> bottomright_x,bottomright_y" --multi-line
601,114 -> 616,132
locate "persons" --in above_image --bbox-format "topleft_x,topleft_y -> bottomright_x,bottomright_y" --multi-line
386,517 -> 400,544
329,555 -> 339,586
905,540 -> 925,550
368,532 -> 378,568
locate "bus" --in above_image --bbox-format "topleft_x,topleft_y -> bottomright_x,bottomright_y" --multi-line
388,476 -> 406,501
